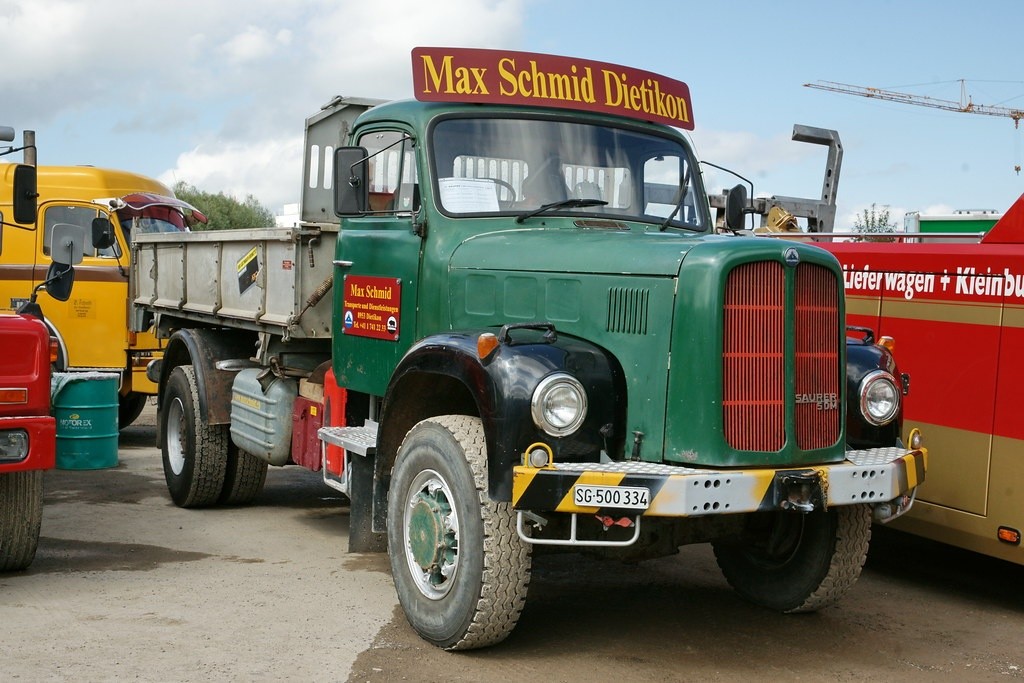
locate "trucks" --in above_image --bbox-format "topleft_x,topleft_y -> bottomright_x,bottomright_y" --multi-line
0,126 -> 86,569
126,94 -> 1024,653
1,162 -> 207,429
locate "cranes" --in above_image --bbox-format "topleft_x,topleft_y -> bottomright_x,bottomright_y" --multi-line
802,80 -> 1024,128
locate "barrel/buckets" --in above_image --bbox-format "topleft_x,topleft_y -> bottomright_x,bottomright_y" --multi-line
50,372 -> 121,472
